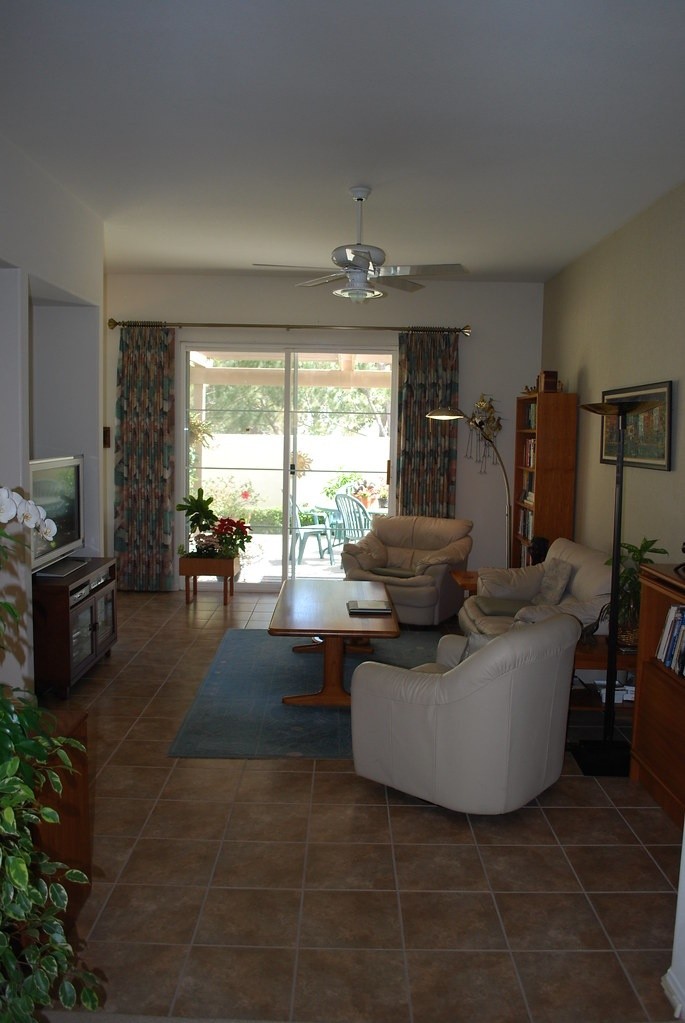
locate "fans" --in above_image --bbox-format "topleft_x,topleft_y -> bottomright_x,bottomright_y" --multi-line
252,186 -> 467,293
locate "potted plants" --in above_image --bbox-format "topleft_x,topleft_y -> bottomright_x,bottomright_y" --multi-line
356,492 -> 371,508
377,493 -> 388,508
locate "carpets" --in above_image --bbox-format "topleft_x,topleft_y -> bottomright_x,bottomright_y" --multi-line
169,628 -> 456,760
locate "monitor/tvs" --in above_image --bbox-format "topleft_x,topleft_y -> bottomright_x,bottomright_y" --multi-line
23,453 -> 90,577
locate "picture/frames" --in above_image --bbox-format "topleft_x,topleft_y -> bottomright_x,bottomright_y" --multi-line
600,380 -> 673,472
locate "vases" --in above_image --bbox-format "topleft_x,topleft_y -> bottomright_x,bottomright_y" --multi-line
178,552 -> 240,606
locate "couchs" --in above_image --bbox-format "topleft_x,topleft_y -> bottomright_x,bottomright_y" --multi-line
341,514 -> 473,625
350,612 -> 582,818
454,537 -> 624,659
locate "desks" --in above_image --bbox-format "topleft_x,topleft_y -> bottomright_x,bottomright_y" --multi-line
315,502 -> 388,557
267,579 -> 401,706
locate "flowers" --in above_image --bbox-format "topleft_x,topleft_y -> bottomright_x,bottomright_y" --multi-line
176,488 -> 253,559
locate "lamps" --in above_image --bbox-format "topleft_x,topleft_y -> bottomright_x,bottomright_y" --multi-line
332,278 -> 386,305
573,398 -> 665,778
426,401 -> 512,568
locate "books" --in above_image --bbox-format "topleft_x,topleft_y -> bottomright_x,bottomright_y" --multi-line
522,470 -> 534,507
537,371 -> 558,393
522,438 -> 535,469
654,604 -> 685,679
521,546 -> 532,568
518,509 -> 532,541
349,600 -> 392,613
524,403 -> 536,429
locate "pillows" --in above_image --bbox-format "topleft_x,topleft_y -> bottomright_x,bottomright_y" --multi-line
533,558 -> 572,605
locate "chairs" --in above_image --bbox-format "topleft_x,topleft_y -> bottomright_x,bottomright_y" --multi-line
281,488 -> 335,566
335,493 -> 373,569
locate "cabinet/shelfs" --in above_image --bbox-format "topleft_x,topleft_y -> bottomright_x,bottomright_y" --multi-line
507,393 -> 579,568
0,709 -> 89,991
629,562 -> 685,831
33,557 -> 118,700
